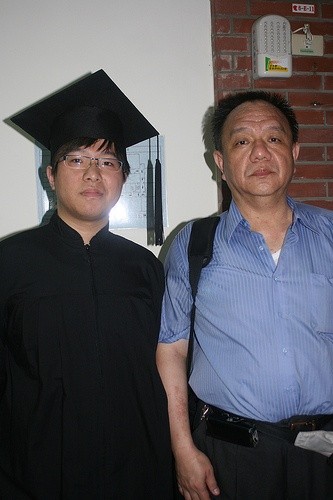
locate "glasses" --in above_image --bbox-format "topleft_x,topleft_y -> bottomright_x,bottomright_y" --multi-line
56,153 -> 127,173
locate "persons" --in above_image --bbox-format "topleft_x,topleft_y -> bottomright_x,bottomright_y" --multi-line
0,69 -> 171,499
155,91 -> 333,499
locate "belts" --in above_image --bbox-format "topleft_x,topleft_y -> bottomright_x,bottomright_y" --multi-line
197,405 -> 333,435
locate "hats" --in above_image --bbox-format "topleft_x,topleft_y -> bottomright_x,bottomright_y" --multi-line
10,68 -> 165,247
